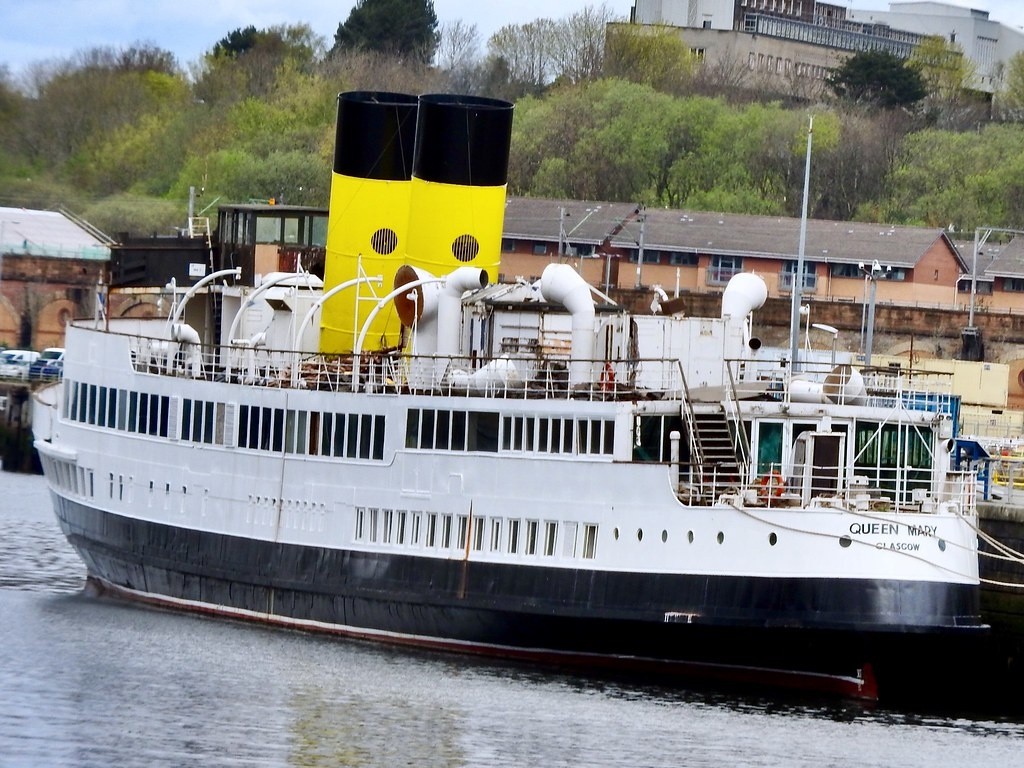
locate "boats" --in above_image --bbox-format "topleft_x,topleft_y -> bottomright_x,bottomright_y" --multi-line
28,89 -> 990,701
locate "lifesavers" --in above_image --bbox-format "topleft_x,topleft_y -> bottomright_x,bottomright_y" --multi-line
761,470 -> 783,501
598,364 -> 614,395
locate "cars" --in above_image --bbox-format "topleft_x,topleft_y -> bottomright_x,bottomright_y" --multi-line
0,348 -> 65,381
